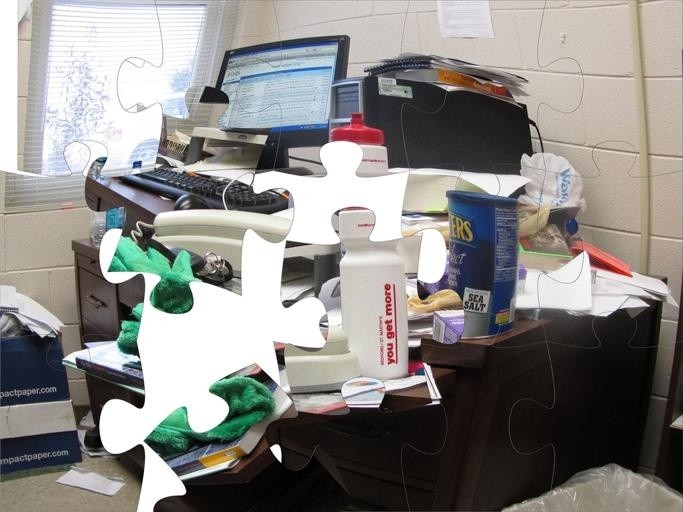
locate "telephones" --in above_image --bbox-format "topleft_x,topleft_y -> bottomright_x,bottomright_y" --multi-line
151,209 -> 293,278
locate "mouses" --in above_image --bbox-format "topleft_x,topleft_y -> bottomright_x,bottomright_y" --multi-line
174,193 -> 208,211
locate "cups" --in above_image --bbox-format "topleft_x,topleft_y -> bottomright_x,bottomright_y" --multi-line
338,211 -> 410,381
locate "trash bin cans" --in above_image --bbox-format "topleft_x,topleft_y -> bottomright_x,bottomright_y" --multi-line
500,462 -> 683,512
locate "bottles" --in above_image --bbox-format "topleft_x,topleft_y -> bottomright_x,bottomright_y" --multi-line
550,214 -> 578,242
131,159 -> 143,174
329,113 -> 391,178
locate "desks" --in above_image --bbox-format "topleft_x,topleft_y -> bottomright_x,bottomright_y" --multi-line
70,156 -> 669,511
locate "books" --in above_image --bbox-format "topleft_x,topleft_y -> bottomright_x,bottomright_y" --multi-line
163,379 -> 293,475
518,222 -> 572,271
75,345 -> 144,386
1,285 -> 19,311
364,50 -> 531,99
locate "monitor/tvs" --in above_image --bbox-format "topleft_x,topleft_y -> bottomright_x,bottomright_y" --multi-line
215,35 -> 350,176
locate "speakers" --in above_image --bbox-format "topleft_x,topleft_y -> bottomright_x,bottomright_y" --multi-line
328,76 -> 365,142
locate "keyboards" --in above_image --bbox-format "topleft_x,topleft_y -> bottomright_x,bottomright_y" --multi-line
119,167 -> 288,214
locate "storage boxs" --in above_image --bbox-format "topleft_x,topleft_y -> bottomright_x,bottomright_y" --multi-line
1,336 -> 84,478
